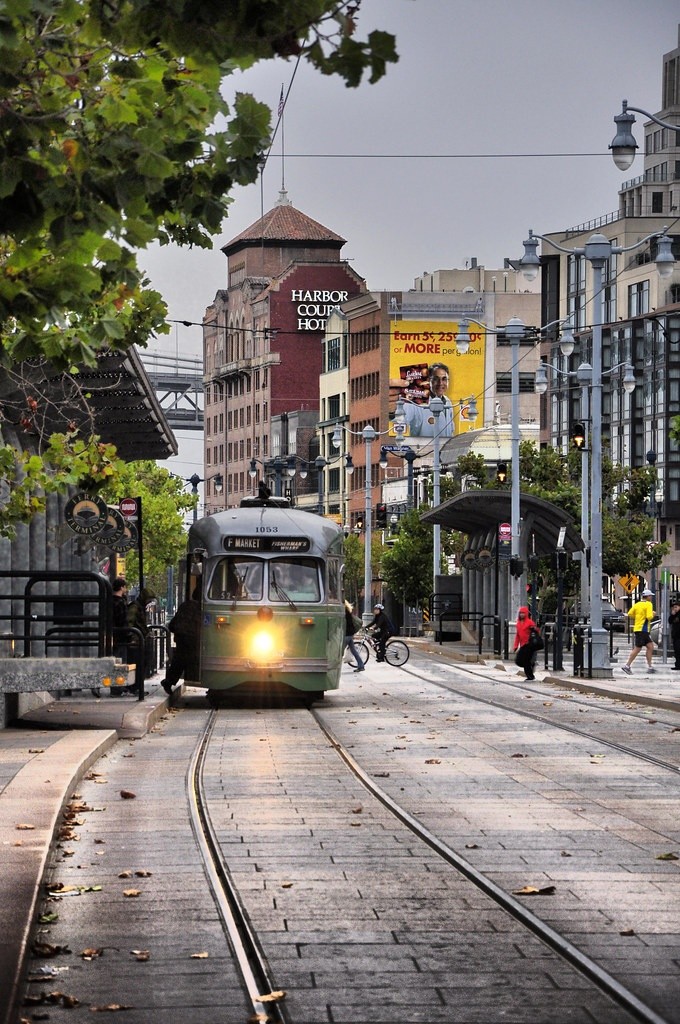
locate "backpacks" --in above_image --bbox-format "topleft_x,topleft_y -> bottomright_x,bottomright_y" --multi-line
529,627 -> 544,652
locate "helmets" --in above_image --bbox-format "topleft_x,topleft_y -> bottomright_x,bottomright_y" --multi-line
374,604 -> 385,610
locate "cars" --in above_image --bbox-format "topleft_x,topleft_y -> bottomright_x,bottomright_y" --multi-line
570,600 -> 626,633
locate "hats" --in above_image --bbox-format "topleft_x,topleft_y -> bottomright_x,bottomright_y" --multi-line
642,589 -> 656,596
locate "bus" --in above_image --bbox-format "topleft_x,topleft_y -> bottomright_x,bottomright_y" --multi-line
182,479 -> 350,703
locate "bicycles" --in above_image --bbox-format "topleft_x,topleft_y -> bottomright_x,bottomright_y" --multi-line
345,627 -> 410,669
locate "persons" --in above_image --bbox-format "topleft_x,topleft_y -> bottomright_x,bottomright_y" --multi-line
513,606 -> 541,682
622,590 -> 658,674
287,562 -> 318,599
668,603 -> 680,670
125,587 -> 156,696
389,361 -> 455,436
362,603 -> 396,662
341,600 -> 365,672
160,587 -> 202,695
110,577 -> 132,695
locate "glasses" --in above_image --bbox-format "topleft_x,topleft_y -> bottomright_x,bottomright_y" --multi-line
430,376 -> 450,384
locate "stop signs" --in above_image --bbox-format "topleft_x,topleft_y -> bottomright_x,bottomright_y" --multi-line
499,523 -> 511,537
118,498 -> 138,516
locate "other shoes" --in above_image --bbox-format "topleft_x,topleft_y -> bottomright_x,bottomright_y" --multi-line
376,659 -> 385,662
648,668 -> 658,673
671,666 -> 680,670
526,676 -> 535,682
109,690 -> 125,698
354,667 -> 365,672
622,666 -> 633,675
160,679 -> 173,695
128,690 -> 149,697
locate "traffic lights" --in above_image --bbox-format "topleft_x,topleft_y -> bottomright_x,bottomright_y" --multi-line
544,551 -> 557,570
527,583 -> 533,594
556,552 -> 568,570
375,502 -> 388,529
356,515 -> 363,529
573,421 -> 586,450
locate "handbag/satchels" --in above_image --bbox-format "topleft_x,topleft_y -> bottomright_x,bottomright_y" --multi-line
351,613 -> 363,632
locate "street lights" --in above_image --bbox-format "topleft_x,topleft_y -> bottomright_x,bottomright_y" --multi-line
330,422 -> 405,628
170,472 -> 223,526
517,228 -> 678,681
378,447 -> 443,638
534,359 -> 638,625
284,453 -> 355,520
453,310 -> 577,665
394,392 -> 479,622
249,454 -> 308,500
